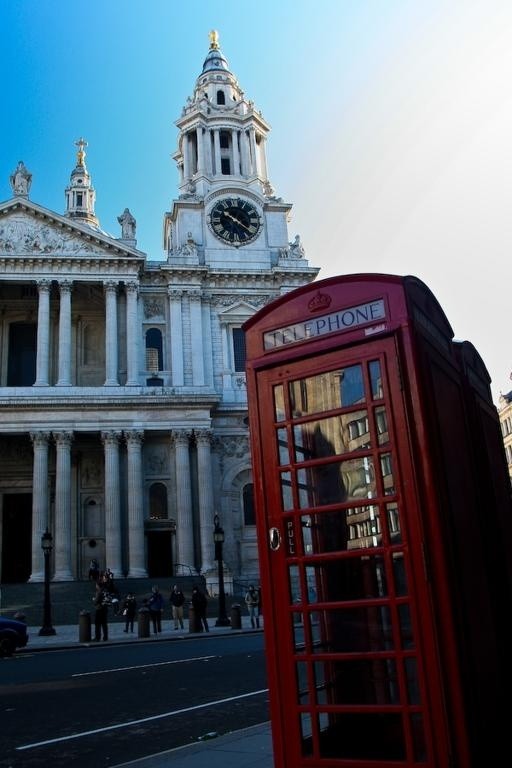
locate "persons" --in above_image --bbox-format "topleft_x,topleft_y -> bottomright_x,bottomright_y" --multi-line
118,207 -> 136,239
185,231 -> 199,256
291,235 -> 304,258
88,557 -> 316,645
12,160 -> 28,194
12,607 -> 28,623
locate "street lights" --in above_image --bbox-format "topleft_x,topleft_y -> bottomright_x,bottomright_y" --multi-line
214,511 -> 231,627
39,526 -> 56,636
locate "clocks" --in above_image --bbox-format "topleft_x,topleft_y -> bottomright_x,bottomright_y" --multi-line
206,196 -> 265,249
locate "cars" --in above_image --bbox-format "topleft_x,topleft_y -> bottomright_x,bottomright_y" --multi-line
0,615 -> 28,657
